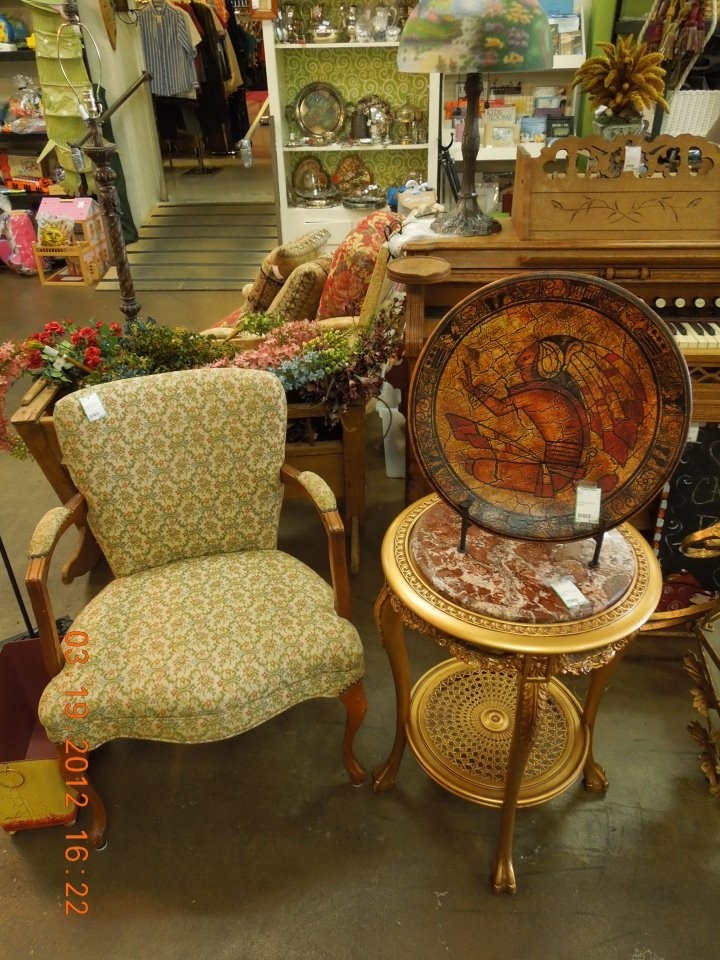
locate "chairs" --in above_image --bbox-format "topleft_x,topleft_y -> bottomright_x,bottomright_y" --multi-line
200,210 -> 404,366
23,367 -> 368,850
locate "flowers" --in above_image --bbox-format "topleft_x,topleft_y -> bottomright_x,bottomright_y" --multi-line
0,314 -> 391,462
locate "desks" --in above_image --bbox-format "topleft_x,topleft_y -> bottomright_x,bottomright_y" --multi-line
368,492 -> 662,894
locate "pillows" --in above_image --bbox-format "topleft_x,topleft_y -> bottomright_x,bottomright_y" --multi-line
238,229 -> 332,322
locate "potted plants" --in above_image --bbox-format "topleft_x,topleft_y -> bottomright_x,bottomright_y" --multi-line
573,34 -> 671,142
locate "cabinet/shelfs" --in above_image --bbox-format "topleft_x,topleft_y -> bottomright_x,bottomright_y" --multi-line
259,0 -> 441,255
